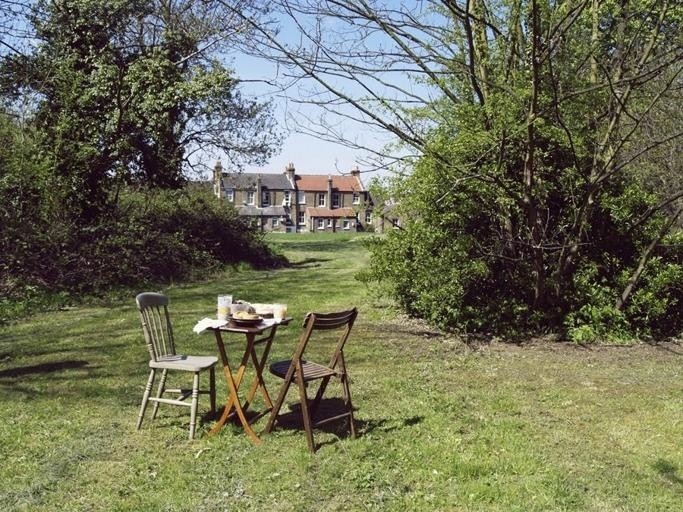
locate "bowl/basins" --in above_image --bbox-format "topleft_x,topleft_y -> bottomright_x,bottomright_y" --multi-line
225,312 -> 265,327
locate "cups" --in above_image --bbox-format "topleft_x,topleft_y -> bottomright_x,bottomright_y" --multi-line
272,302 -> 288,320
217,293 -> 233,317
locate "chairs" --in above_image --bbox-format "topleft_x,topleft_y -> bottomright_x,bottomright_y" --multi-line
134,292 -> 218,442
265,305 -> 359,453
